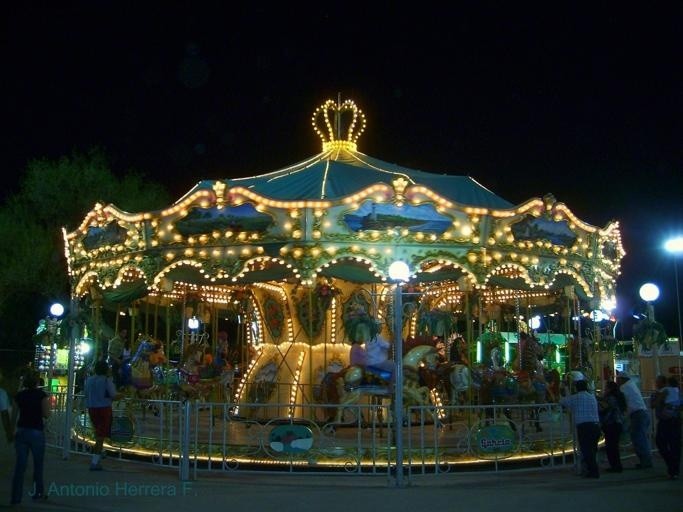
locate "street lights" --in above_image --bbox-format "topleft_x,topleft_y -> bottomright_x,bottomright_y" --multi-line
661,234 -> 682,350
187,318 -> 199,343
47,302 -> 64,391
387,258 -> 410,487
639,283 -> 659,324
589,310 -> 602,342
528,317 -> 541,337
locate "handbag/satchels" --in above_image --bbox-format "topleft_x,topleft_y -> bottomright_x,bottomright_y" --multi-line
105,390 -> 111,398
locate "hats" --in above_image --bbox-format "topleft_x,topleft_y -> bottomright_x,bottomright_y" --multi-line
616,371 -> 628,378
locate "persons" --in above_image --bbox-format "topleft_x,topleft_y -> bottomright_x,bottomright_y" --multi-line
107,327 -> 128,389
8,369 -> 51,505
650,375 -> 679,479
558,378 -> 601,480
594,380 -> 628,472
83,361 -> 122,472
658,377 -> 682,418
616,376 -> 653,468
0,387 -> 13,443
344,334 -> 391,367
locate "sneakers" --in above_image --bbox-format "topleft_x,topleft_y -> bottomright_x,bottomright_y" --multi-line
89,464 -> 101,471
